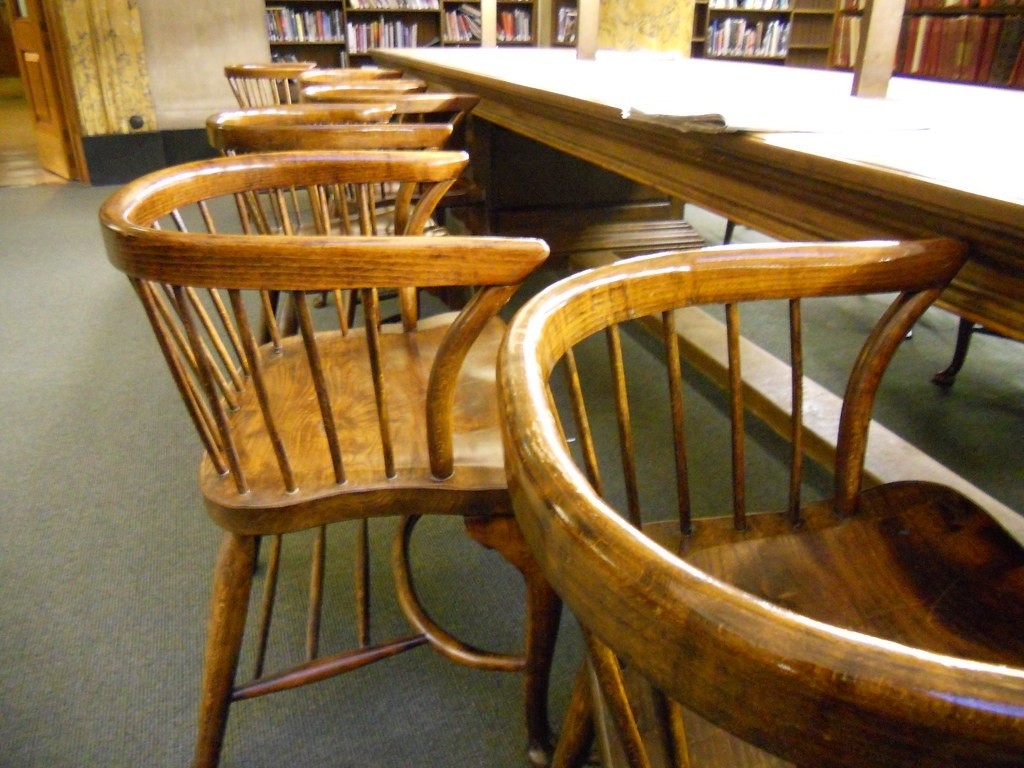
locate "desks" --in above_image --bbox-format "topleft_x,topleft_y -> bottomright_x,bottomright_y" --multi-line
366,45 -> 1024,559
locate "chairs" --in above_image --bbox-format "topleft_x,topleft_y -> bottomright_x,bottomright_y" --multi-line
498,234 -> 1024,768
206,61 -> 495,344
97,151 -> 566,768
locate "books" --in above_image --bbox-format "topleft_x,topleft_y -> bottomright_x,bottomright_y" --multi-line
266,0 -> 579,71
702,0 -> 1023,86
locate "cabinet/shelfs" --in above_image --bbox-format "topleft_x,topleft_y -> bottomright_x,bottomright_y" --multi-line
266,0 -> 580,123
693,1 -> 1023,93
